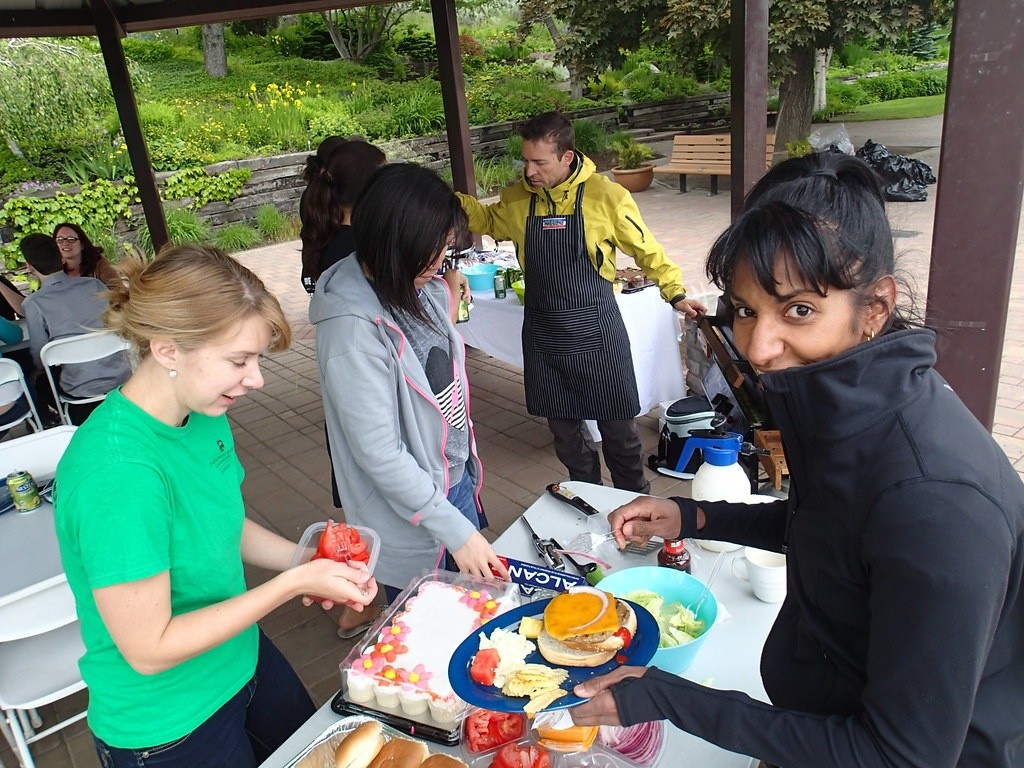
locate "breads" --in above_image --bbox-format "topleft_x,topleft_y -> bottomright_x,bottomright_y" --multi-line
335,721 -> 469,768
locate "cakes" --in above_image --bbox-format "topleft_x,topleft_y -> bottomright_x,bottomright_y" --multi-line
344,580 -> 517,724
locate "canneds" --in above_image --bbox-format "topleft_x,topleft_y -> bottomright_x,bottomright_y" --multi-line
494,265 -> 525,299
455,284 -> 469,324
6,468 -> 43,514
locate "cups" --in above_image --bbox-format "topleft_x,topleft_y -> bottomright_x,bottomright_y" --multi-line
732,547 -> 787,604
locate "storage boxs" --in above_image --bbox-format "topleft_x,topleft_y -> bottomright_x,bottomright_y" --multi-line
337,567 -> 521,725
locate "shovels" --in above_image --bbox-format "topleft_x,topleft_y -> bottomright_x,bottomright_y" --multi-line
546,483 -> 663,556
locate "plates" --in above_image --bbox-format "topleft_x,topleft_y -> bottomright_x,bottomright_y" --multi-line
449,598 -> 660,714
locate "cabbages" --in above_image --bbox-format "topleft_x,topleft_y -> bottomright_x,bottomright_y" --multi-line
616,591 -> 704,648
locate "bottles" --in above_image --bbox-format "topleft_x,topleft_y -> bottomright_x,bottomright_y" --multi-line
494,268 -> 507,298
457,285 -> 469,324
658,539 -> 692,575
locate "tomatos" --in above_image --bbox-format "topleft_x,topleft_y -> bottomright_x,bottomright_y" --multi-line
470,649 -> 500,686
466,708 -> 551,768
307,518 -> 369,604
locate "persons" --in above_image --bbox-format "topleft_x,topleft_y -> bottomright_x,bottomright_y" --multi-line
19,233 -> 133,425
0,315 -> 35,425
0,274 -> 26,321
299,136 -> 388,639
53,223 -> 125,290
453,111 -> 708,496
50,244 -> 378,768
574,151 -> 1024,768
308,164 -> 512,606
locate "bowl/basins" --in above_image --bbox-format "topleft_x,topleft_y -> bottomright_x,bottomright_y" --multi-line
592,564 -> 718,675
461,262 -> 503,291
511,280 -> 525,306
289,521 -> 381,605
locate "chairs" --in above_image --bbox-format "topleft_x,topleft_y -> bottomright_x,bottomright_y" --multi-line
0,326 -> 136,768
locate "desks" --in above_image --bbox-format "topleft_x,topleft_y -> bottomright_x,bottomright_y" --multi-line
0,471 -> 66,597
0,318 -> 33,354
255,478 -> 786,766
453,259 -> 680,442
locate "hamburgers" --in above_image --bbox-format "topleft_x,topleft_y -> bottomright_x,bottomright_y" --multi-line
537,585 -> 637,668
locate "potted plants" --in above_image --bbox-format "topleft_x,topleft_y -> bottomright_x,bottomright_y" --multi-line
604,136 -> 656,195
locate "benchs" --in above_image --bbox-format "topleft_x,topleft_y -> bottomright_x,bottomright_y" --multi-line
651,134 -> 776,196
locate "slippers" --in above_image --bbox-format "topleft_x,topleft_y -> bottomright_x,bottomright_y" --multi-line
338,604 -> 389,640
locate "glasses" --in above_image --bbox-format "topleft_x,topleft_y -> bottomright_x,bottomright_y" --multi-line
55,236 -> 81,244
